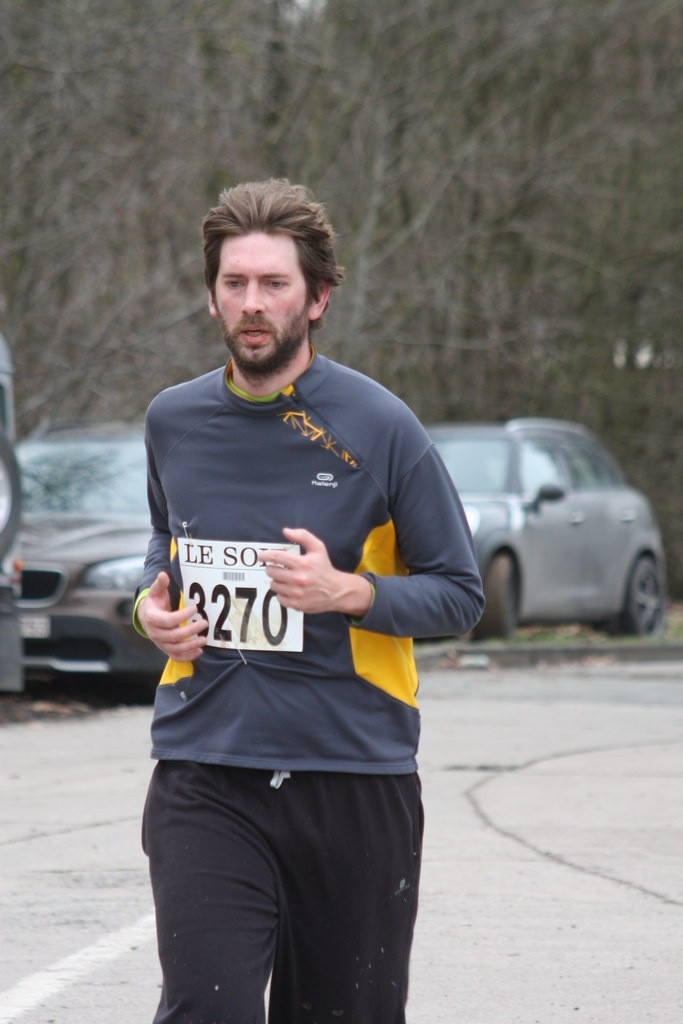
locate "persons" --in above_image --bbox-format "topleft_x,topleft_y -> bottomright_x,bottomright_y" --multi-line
132,178 -> 486,1024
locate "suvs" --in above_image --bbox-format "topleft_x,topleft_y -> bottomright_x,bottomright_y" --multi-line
416,416 -> 669,641
0,327 -> 38,700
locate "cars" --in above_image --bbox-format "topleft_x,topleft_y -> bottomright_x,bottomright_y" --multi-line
1,416 -> 171,704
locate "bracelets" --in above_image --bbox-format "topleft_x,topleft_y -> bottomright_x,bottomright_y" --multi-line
132,588 -> 150,639
